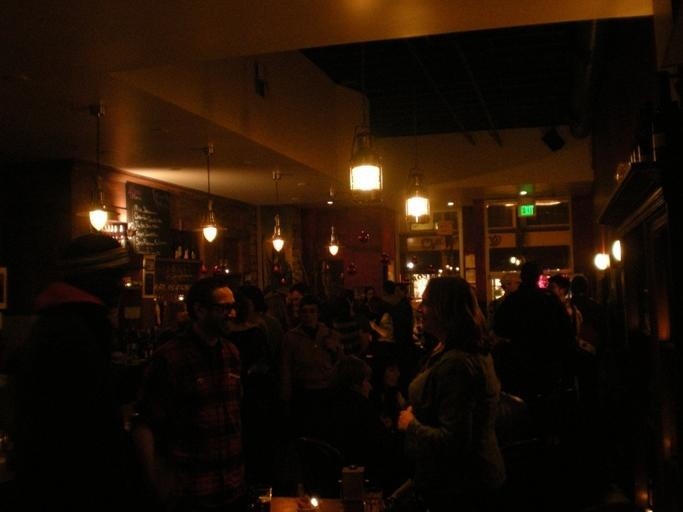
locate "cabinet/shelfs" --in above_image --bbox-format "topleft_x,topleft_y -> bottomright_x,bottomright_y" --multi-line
395,206 -> 465,284
483,196 -> 574,320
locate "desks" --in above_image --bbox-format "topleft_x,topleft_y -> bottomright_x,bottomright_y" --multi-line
269,496 -> 344,512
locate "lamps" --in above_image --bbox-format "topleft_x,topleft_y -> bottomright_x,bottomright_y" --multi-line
402,105 -> 431,224
201,144 -> 220,245
270,168 -> 285,253
347,105 -> 385,194
88,104 -> 112,234
328,210 -> 339,256
327,184 -> 336,205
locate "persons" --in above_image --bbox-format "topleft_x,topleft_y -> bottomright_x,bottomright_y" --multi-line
1,223 -> 640,509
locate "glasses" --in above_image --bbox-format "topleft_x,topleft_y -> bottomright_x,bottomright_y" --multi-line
211,303 -> 234,311
